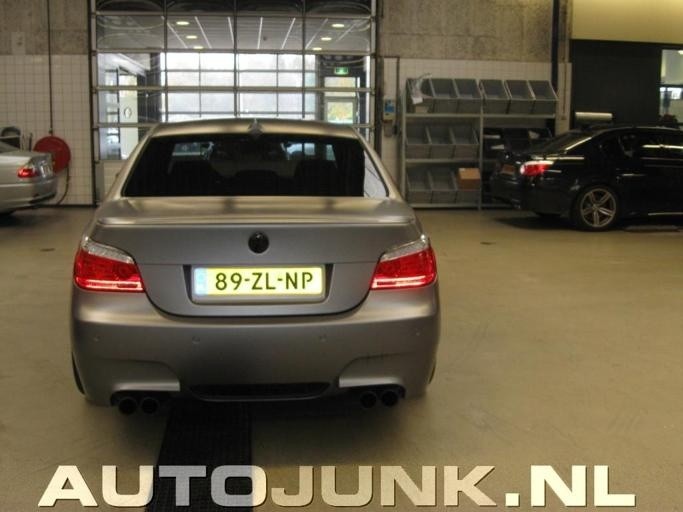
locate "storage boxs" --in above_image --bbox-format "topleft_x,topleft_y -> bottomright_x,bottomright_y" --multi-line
398,76 -> 559,208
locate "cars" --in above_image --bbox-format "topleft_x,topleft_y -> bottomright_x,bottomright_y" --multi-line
0,138 -> 57,217
63,114 -> 446,417
489,119 -> 683,230
